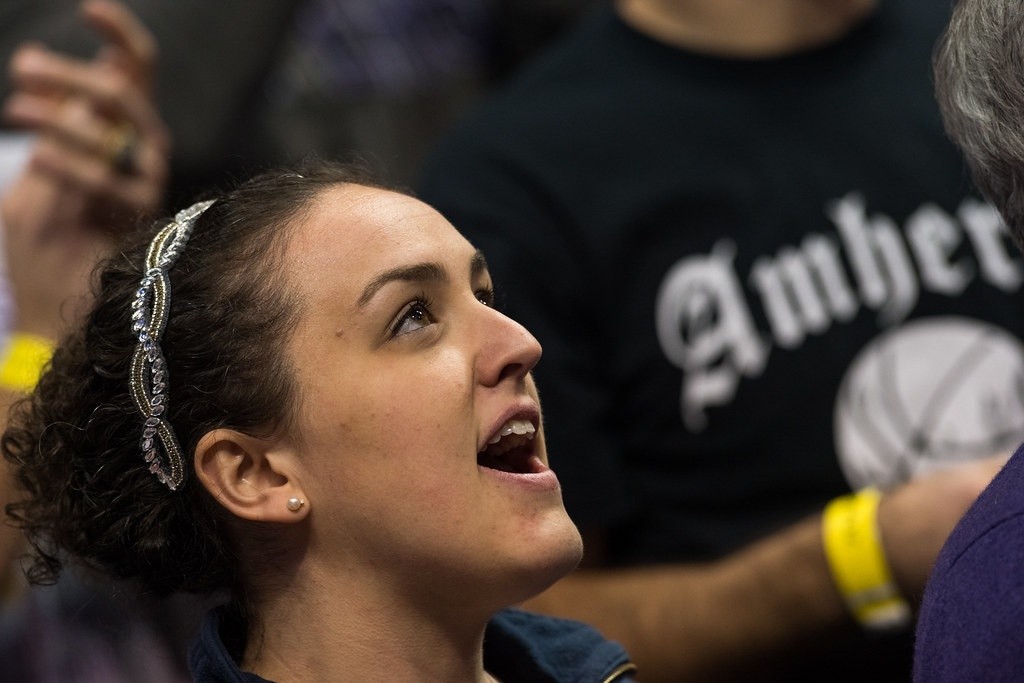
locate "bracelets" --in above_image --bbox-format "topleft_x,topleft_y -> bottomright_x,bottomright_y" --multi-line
821,485 -> 910,629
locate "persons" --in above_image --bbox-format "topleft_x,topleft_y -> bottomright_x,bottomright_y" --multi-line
915,3 -> 1024,683
230,0 -> 1024,683
1,167 -> 643,683
1,3 -> 157,612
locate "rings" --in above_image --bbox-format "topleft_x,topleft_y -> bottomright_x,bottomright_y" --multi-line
105,123 -> 142,175
